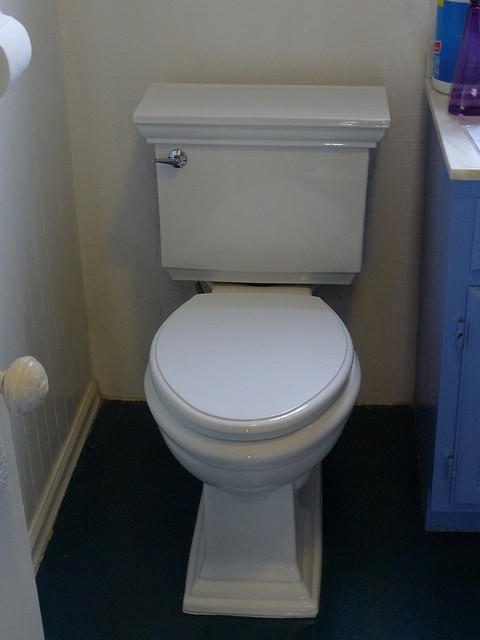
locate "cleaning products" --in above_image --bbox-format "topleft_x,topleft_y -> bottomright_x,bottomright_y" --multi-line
431,1 -> 468,94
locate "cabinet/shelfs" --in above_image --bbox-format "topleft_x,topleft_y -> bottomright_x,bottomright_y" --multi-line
414,77 -> 480,532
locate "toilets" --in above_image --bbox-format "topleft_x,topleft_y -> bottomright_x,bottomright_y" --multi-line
133,80 -> 393,619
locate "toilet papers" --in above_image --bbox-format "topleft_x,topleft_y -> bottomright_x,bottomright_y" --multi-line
0,9 -> 34,101
